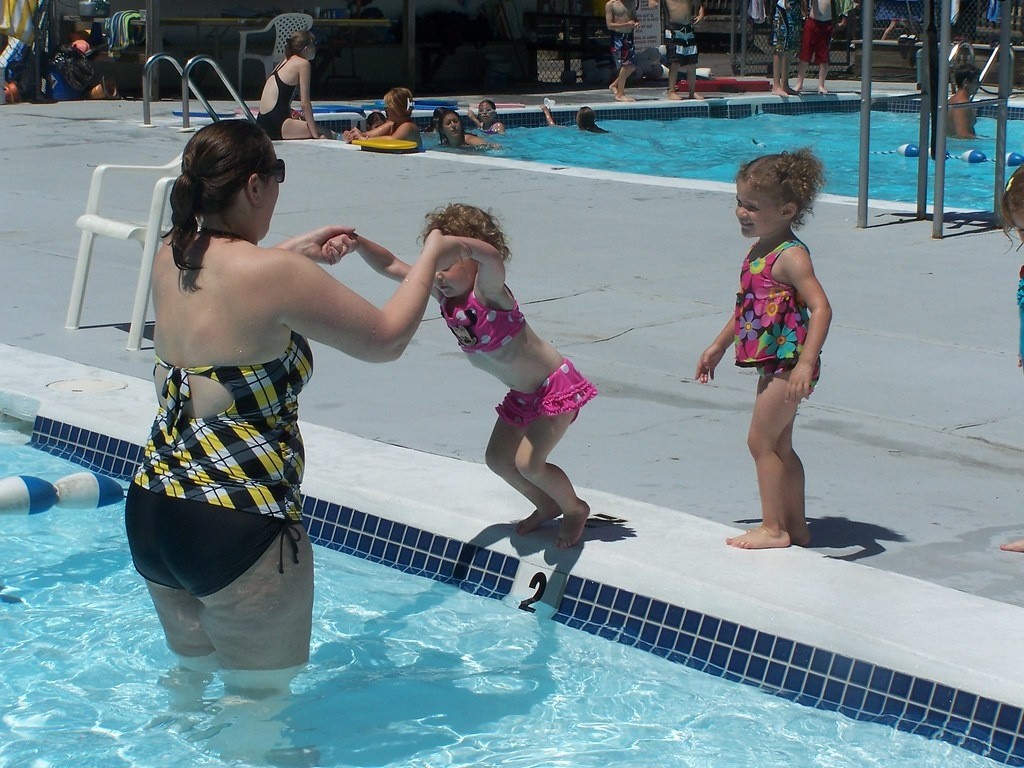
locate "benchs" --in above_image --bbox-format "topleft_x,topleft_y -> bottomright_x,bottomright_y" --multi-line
850,40 -> 1024,86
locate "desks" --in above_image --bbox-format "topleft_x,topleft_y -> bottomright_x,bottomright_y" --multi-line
61,14 -> 392,98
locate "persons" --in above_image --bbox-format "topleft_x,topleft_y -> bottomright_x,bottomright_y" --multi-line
605,0 -> 641,101
943,63 -> 981,139
342,87 -> 424,148
322,203 -> 599,548
695,143 -> 833,550
468,99 -> 505,134
122,117 -> 460,673
762,0 -> 806,97
1001,158 -> 1024,556
437,110 -> 503,150
420,107 -> 448,132
365,111 -> 386,133
792,0 -> 853,93
540,103 -> 609,134
255,30 -> 340,142
649,0 -> 706,100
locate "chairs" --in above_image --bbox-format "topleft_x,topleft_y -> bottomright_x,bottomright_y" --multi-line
64,148 -> 202,351
237,12 -> 313,100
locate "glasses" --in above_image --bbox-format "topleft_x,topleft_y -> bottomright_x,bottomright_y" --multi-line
314,41 -> 318,46
259,158 -> 286,184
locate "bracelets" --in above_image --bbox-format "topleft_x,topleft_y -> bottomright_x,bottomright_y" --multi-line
548,121 -> 554,125
